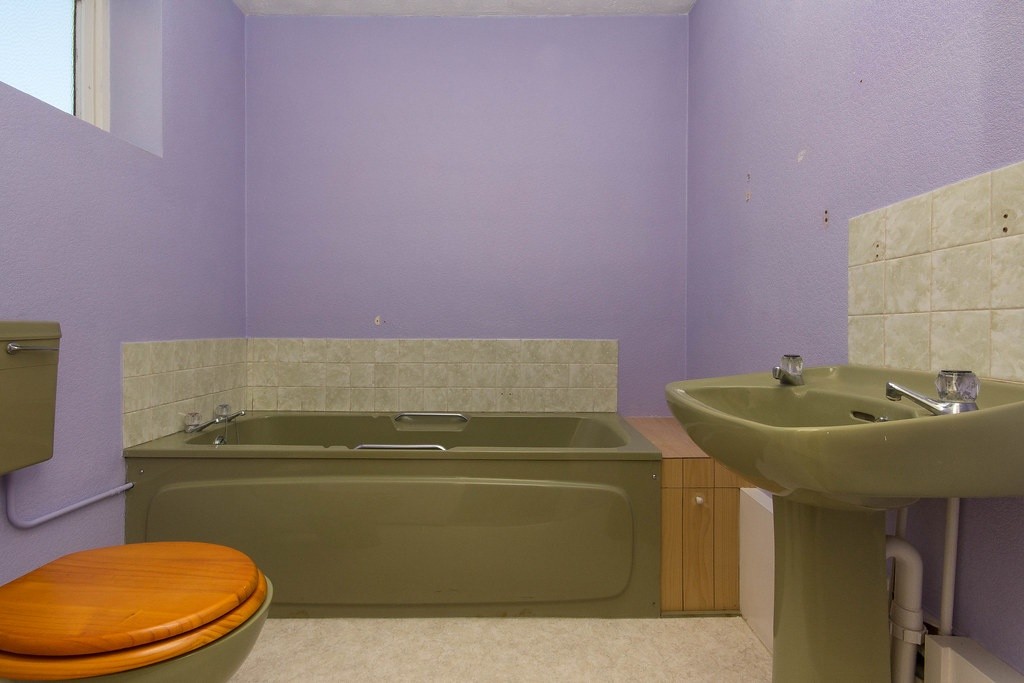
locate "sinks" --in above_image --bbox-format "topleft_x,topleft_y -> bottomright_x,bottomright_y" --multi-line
661,370 -> 1024,509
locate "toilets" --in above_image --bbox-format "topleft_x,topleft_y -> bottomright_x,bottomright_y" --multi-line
0,538 -> 276,683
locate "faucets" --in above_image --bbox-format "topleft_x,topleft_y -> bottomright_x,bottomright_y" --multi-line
886,365 -> 982,416
215,403 -> 246,423
183,412 -> 220,434
772,351 -> 804,385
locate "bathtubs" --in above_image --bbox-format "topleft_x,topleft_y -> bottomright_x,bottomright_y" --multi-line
120,406 -> 666,621
